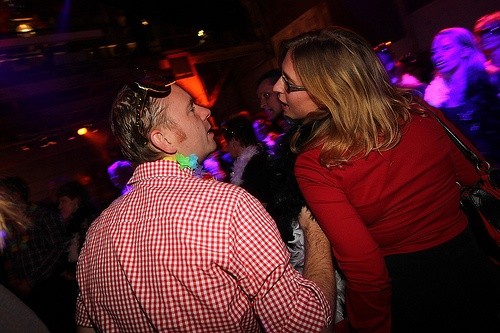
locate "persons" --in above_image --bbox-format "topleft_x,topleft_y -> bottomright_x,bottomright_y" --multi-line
373,7 -> 500,166
204,68 -> 348,333
0,159 -> 135,333
75,67 -> 338,333
273,27 -> 500,333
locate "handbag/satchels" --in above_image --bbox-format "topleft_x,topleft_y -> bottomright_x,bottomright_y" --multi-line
403,91 -> 499,268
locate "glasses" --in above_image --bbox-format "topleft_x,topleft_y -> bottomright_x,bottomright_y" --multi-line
130,67 -> 171,135
281,75 -> 307,94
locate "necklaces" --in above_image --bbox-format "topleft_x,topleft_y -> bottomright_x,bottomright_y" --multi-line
230,144 -> 263,187
161,154 -> 210,179
